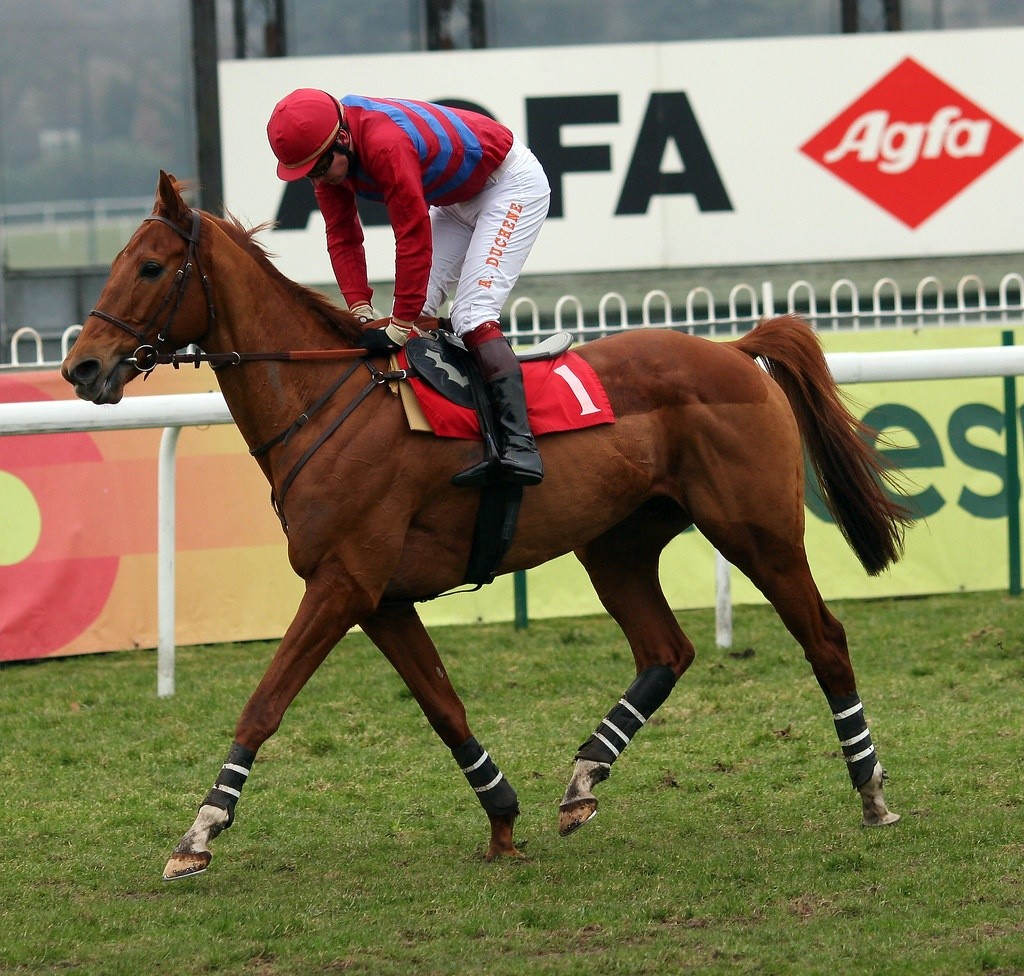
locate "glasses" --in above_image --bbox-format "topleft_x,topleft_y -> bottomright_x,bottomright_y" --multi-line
305,142 -> 337,178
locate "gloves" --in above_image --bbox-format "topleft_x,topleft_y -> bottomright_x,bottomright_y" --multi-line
354,319 -> 403,361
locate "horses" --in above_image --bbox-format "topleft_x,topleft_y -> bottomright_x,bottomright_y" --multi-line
59,170 -> 915,881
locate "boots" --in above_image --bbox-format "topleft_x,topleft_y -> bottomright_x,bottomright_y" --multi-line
450,336 -> 544,488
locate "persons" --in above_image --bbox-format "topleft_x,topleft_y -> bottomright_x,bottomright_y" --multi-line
265,87 -> 551,491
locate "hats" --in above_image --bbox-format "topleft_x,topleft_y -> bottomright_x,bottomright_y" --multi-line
266,88 -> 344,182
